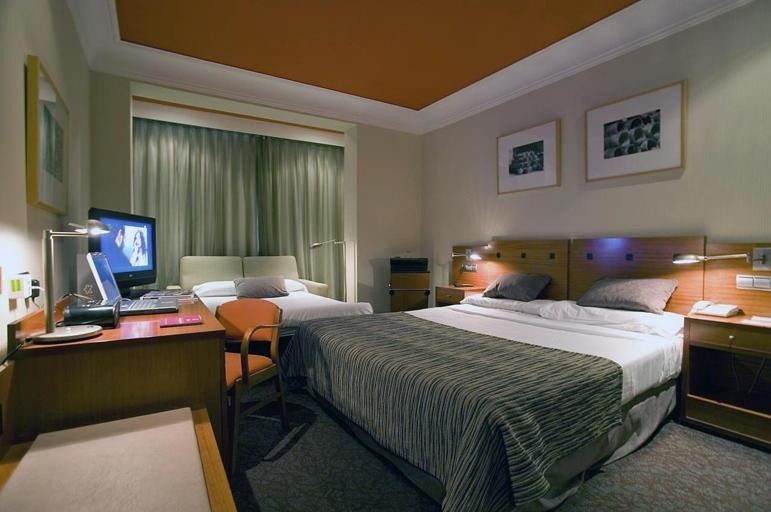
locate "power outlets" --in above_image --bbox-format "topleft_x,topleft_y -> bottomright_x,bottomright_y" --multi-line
23,275 -> 33,298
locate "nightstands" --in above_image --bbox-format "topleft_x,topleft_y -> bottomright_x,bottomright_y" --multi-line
681,314 -> 771,453
435,286 -> 485,308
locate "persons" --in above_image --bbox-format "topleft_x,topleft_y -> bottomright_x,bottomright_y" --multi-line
103,224 -> 131,270
129,231 -> 150,268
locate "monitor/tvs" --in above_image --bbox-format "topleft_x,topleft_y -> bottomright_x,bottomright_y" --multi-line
87,207 -> 161,299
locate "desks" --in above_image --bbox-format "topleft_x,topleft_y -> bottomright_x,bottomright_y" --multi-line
6,290 -> 229,466
1,407 -> 236,511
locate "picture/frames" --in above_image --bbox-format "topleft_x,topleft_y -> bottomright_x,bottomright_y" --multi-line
495,119 -> 562,194
28,54 -> 68,216
585,80 -> 687,181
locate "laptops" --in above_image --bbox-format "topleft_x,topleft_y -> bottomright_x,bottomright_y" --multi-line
86,251 -> 179,316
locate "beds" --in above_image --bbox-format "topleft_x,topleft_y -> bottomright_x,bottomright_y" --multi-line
166,255 -> 373,344
282,236 -> 710,512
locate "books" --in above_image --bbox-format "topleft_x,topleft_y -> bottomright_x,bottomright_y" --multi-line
159,313 -> 202,328
139,290 -> 195,303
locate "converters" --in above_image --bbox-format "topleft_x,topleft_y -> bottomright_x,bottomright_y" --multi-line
31,279 -> 40,298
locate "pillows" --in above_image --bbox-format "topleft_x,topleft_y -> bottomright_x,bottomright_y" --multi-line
577,276 -> 679,315
484,274 -> 553,303
234,276 -> 290,299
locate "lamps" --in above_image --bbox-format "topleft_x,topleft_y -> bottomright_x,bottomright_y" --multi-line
451,252 -> 482,260
310,239 -> 346,248
674,251 -> 766,264
36,218 -> 108,342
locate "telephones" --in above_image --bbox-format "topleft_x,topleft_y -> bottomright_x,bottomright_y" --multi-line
690,301 -> 739,317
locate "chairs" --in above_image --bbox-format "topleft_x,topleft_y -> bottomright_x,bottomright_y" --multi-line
215,299 -> 284,481
